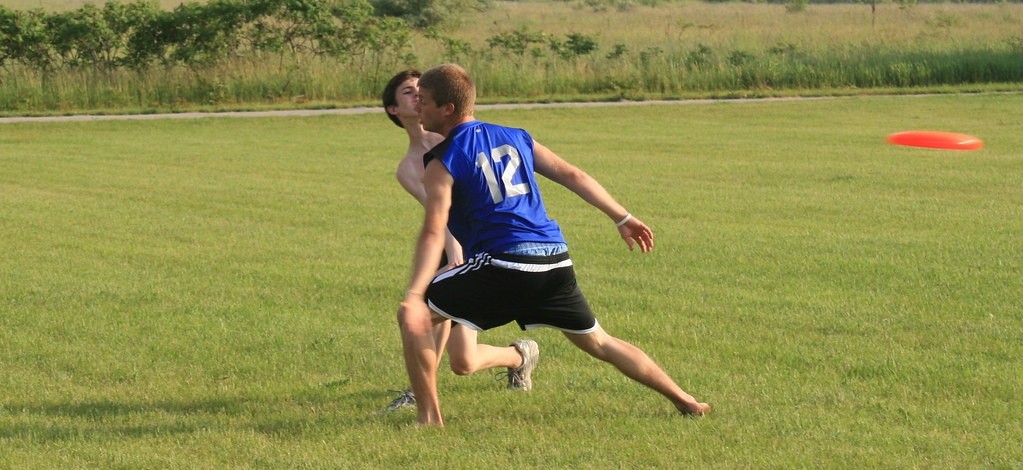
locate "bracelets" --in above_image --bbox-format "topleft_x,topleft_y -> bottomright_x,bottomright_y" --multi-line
616,213 -> 632,227
405,290 -> 424,298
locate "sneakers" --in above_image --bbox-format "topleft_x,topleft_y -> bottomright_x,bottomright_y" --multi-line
495,339 -> 539,391
372,388 -> 416,414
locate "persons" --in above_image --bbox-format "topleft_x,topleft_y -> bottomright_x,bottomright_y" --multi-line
381,65 -> 711,427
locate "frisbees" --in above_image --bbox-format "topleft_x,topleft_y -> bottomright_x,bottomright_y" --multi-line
885,130 -> 982,151
